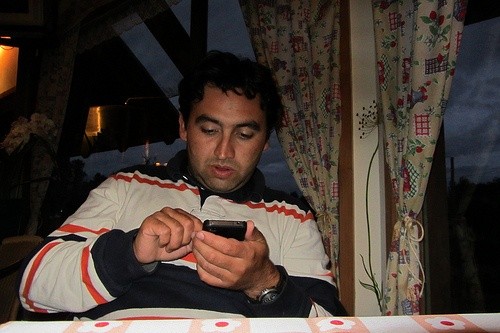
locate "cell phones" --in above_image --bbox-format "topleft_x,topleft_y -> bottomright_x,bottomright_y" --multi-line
202,220 -> 248,242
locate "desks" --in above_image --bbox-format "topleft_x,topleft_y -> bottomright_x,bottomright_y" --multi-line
0,312 -> 500,333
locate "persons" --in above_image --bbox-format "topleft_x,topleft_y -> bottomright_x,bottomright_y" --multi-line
17,50 -> 354,317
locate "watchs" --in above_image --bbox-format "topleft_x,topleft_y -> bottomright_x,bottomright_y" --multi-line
248,271 -> 285,305
